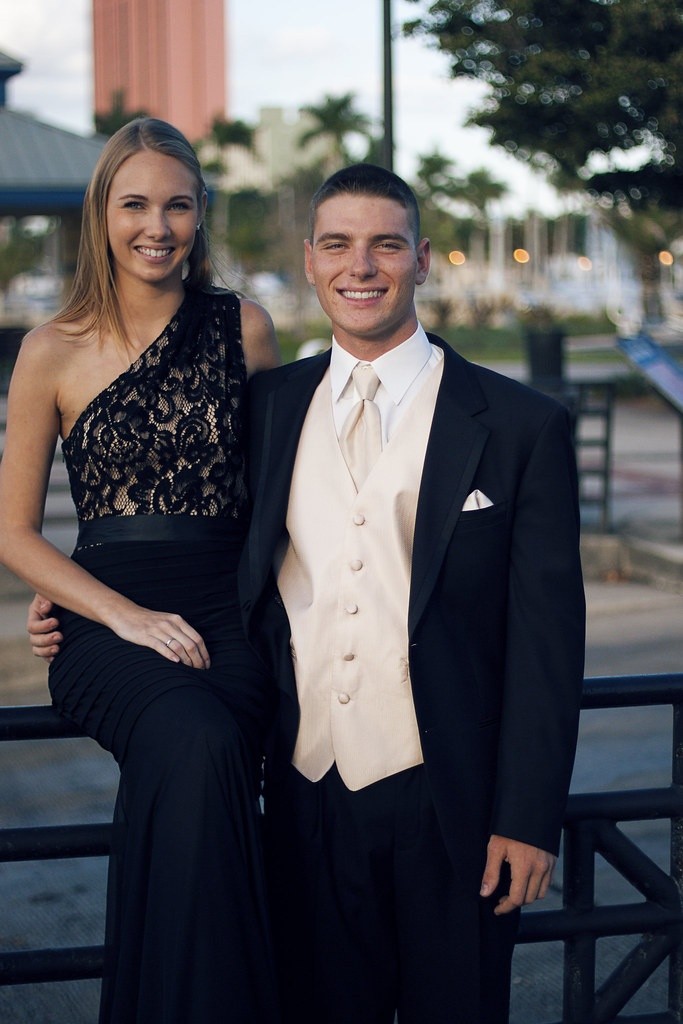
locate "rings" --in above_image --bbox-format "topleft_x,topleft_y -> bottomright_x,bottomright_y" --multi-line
165,637 -> 175,646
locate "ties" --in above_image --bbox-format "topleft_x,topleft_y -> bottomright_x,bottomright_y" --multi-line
339,364 -> 380,494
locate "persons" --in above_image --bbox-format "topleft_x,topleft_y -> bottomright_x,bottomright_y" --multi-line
24,164 -> 583,1023
1,118 -> 282,1023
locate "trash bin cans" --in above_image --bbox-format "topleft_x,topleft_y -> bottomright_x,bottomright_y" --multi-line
523,330 -> 568,383
